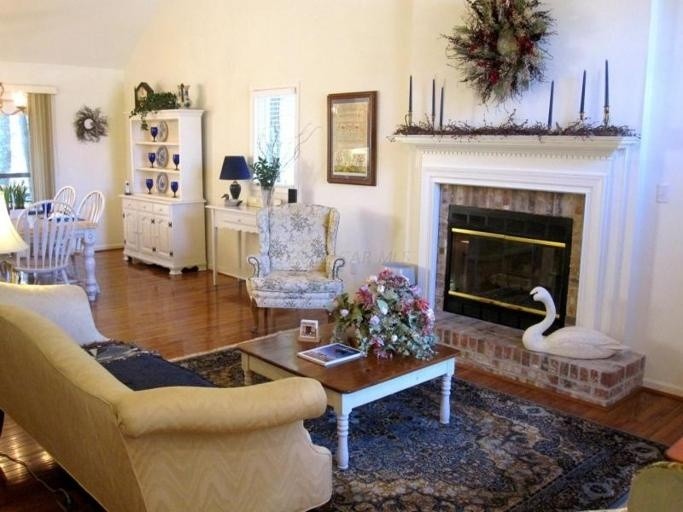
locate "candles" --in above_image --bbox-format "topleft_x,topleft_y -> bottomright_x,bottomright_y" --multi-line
605,60 -> 609,106
432,79 -> 435,115
440,87 -> 443,128
580,70 -> 586,113
547,81 -> 554,128
409,75 -> 412,112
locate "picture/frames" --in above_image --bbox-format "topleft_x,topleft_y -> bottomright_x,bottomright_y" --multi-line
326,91 -> 377,185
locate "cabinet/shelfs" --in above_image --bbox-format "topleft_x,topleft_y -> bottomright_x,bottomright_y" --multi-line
129,109 -> 206,201
119,194 -> 207,275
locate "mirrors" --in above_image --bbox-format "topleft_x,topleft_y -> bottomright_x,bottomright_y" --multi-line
249,86 -> 301,192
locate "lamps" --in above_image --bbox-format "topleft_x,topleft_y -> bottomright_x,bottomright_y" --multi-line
219,156 -> 250,199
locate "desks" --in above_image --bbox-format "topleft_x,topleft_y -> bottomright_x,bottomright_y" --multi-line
205,204 -> 257,299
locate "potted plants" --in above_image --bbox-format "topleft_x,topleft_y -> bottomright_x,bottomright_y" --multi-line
249,156 -> 282,206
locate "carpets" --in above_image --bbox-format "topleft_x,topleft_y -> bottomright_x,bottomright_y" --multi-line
169,328 -> 668,512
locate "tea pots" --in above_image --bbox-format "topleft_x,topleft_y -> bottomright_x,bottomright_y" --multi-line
177,84 -> 192,108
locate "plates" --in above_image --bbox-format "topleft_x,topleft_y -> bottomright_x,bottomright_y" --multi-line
156,120 -> 168,193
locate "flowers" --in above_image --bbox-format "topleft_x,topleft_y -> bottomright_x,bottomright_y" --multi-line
329,269 -> 438,363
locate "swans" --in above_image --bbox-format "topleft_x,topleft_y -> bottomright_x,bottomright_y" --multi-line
519,286 -> 630,359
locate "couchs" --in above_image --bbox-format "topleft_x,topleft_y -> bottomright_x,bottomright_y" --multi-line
0,280 -> 333,512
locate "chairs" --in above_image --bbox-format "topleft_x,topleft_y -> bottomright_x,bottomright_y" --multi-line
0,185 -> 104,301
245,202 -> 347,334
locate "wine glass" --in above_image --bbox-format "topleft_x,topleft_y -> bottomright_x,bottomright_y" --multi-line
146,179 -> 153,194
148,152 -> 155,167
42,202 -> 51,217
150,126 -> 157,142
171,182 -> 178,198
173,154 -> 179,170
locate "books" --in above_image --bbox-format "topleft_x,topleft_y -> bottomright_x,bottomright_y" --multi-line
296,342 -> 366,367
